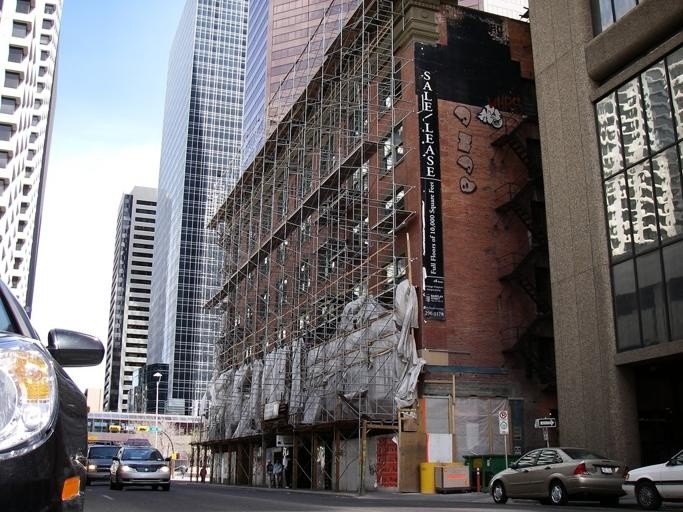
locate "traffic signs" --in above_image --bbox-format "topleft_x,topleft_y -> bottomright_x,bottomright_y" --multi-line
537,418 -> 555,428
149,426 -> 161,432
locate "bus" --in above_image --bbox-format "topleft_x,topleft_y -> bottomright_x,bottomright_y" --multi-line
109,437 -> 171,492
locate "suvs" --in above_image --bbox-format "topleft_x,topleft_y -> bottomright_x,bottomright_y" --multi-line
87,442 -> 122,486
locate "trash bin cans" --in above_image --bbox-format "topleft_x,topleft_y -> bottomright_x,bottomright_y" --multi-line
419,462 -> 440,494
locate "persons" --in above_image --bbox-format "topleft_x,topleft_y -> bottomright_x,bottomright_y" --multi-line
272,459 -> 282,488
180,463 -> 186,479
266,459 -> 273,487
200,465 -> 206,482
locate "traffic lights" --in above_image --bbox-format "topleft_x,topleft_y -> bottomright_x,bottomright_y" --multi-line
175,454 -> 180,459
137,426 -> 149,432
108,425 -> 121,432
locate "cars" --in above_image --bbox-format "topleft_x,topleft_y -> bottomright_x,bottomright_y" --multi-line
486,446 -> 626,507
0,276 -> 109,512
622,447 -> 683,508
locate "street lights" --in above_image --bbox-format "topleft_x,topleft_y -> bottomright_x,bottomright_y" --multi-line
152,370 -> 163,448
